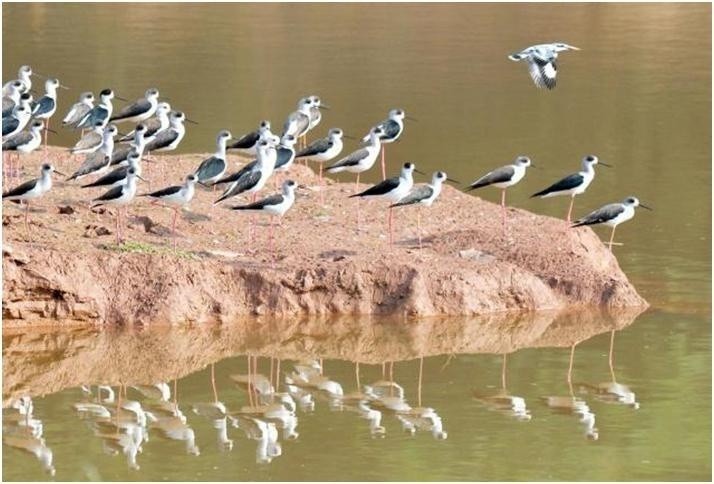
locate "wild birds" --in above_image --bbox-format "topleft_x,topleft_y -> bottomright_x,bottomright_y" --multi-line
540,341 -> 605,448
391,169 -> 448,227
574,197 -> 655,241
466,152 -> 536,214
3,347 -> 451,477
349,161 -> 418,217
299,123 -> 345,171
328,127 -> 384,189
582,327 -> 642,412
473,348 -> 532,420
527,152 -> 616,224
502,41 -> 582,92
366,106 -> 408,157
3,65 -> 319,228
